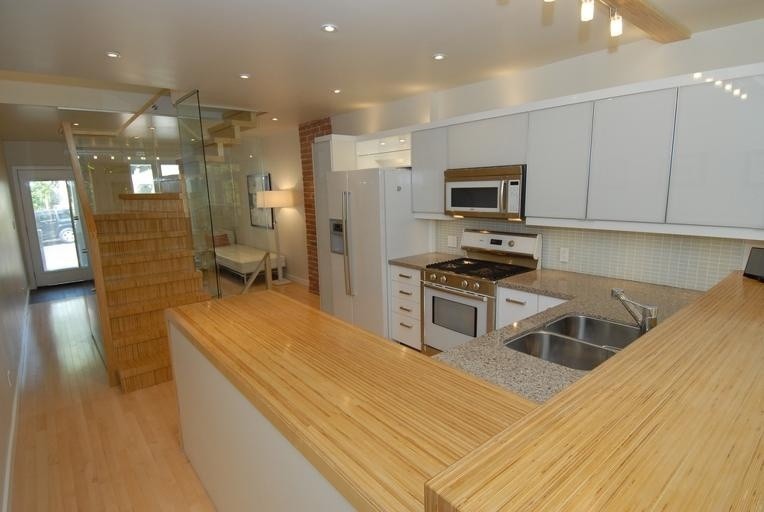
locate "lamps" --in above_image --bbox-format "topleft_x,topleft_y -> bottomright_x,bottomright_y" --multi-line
256,191 -> 303,285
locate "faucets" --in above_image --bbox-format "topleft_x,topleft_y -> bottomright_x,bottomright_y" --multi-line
610,286 -> 659,336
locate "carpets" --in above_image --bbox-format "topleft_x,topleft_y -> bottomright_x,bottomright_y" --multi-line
27,278 -> 96,305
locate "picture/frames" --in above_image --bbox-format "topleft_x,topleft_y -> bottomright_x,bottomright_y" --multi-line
246,171 -> 275,231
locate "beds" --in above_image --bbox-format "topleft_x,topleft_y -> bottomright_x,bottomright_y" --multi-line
209,229 -> 286,285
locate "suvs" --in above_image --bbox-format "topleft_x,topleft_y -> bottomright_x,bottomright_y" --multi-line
35,210 -> 75,243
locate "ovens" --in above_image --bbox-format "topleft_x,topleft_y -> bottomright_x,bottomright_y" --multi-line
421,269 -> 495,352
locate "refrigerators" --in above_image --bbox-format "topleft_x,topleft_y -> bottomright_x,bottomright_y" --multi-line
326,168 -> 435,340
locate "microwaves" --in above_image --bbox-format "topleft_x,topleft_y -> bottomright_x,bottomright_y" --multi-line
444,165 -> 525,222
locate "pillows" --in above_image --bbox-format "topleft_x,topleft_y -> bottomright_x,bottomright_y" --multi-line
206,233 -> 230,247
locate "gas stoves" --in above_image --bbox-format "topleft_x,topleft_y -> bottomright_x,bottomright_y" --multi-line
425,257 -> 535,281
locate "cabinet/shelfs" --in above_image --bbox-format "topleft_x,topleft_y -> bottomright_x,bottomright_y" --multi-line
411,119 -> 449,218
352,131 -> 411,172
448,104 -> 526,170
496,283 -> 569,331
525,89 -> 594,228
585,75 -> 674,234
663,62 -> 763,242
388,262 -> 424,352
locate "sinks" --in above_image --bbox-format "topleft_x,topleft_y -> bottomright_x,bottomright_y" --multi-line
546,315 -> 641,350
503,332 -> 616,371
